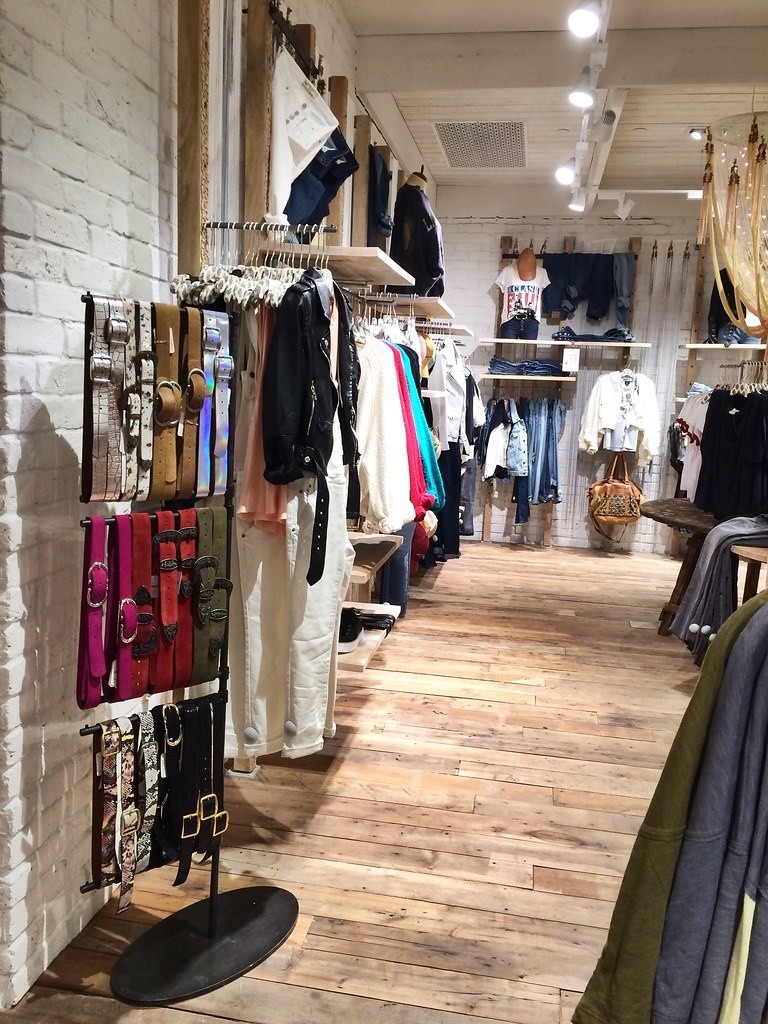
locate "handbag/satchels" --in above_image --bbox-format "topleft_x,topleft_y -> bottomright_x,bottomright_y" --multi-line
588,450 -> 641,543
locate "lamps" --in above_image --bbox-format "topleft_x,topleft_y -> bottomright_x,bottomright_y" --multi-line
591,109 -> 616,144
555,158 -> 575,186
567,0 -> 601,39
614,197 -> 636,221
568,193 -> 586,211
691,130 -> 704,140
567,70 -> 594,107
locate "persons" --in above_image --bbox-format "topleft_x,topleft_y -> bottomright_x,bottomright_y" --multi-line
392,172 -> 447,298
707,268 -> 762,343
494,248 -> 550,340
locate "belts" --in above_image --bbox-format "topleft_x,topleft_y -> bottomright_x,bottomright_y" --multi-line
76,294 -> 235,894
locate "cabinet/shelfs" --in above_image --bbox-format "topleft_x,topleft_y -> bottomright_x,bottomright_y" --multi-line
479,337 -> 652,381
673,344 -> 768,403
258,242 -> 415,673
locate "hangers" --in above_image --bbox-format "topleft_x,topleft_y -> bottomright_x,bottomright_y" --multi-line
169,221 -> 334,316
700,359 -> 768,404
620,355 -> 634,382
421,320 -> 471,368
349,288 -> 418,345
490,385 -> 561,405
